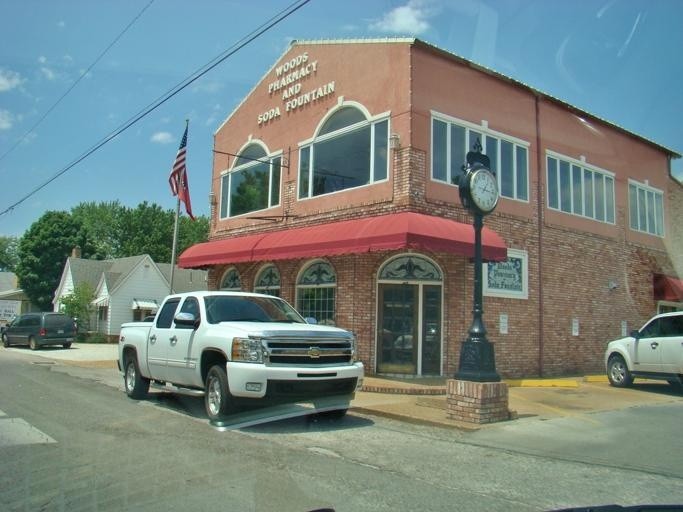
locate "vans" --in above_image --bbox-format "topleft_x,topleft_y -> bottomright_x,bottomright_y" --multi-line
2,312 -> 76,350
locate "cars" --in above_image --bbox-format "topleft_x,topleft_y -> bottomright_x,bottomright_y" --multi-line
604,311 -> 683,387
393,323 -> 439,362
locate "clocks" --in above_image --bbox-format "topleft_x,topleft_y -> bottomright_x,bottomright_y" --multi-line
472,170 -> 498,212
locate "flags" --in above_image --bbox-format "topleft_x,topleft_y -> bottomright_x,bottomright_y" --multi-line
167,124 -> 195,223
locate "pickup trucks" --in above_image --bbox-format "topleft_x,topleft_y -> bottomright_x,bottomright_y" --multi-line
117,291 -> 365,421
